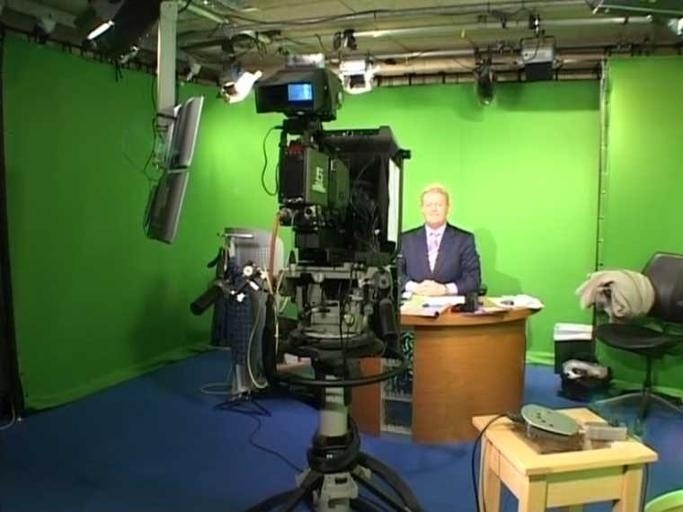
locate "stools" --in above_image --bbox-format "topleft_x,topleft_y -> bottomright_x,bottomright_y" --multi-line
470,406 -> 659,512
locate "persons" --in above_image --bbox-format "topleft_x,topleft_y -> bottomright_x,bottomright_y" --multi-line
401,187 -> 482,298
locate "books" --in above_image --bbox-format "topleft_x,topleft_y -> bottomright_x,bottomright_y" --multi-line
553,322 -> 593,341
401,291 -> 545,318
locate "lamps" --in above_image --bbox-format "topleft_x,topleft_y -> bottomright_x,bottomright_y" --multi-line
75,5 -> 116,41
472,63 -> 498,103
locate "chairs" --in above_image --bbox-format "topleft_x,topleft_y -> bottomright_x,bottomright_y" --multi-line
596,251 -> 683,436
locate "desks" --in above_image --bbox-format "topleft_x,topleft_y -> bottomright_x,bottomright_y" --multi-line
350,295 -> 542,443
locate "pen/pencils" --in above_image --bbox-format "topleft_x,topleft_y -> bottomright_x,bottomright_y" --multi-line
422,303 -> 444,307
501,301 -> 514,305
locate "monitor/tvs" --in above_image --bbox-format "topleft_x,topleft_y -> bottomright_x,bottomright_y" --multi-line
172,95 -> 205,167
145,170 -> 189,244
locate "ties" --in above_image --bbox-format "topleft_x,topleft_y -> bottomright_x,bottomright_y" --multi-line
427,231 -> 440,274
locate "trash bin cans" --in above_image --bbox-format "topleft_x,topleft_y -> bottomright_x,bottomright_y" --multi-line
554,323 -> 596,374
